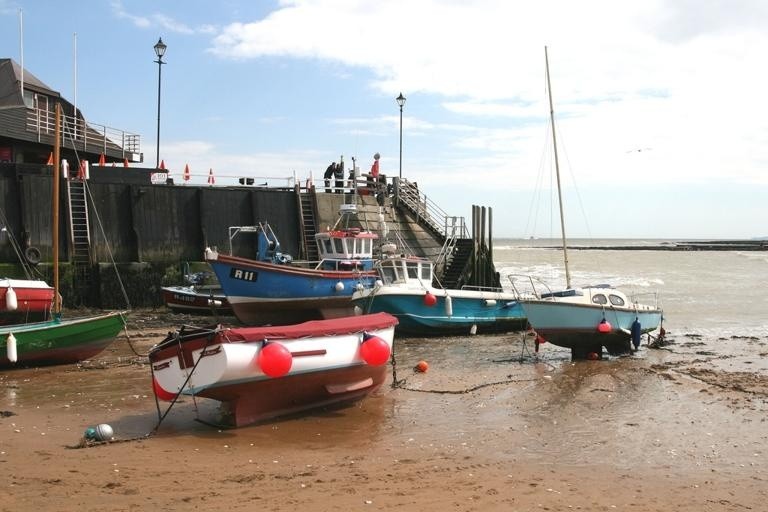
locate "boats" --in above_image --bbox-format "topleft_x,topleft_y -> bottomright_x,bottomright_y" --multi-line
149,312 -> 399,430
351,243 -> 536,337
204,203 -> 378,325
0,279 -> 56,324
161,285 -> 233,315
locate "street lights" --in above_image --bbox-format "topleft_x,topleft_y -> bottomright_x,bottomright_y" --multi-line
153,36 -> 167,169
395,92 -> 407,179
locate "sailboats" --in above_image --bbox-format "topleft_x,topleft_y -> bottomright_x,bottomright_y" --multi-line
0,102 -> 131,369
507,45 -> 662,360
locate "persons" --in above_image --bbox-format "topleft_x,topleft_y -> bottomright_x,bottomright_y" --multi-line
324,162 -> 336,193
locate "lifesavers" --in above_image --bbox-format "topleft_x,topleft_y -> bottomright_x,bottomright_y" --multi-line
343,228 -> 361,234
340,261 -> 362,268
